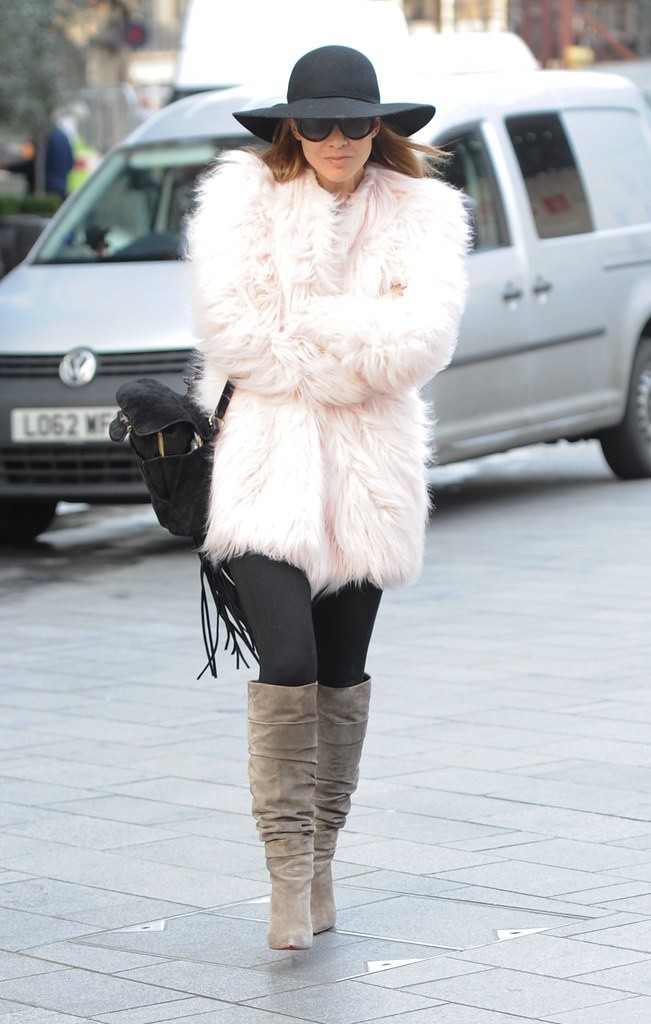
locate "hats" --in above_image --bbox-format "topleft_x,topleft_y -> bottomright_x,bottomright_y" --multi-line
232,45 -> 436,144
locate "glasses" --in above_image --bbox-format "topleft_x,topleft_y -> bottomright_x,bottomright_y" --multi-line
296,117 -> 375,143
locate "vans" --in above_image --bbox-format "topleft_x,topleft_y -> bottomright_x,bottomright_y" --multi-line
0,63 -> 649,555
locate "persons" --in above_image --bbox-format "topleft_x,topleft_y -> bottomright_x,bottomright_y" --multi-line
44,102 -> 86,250
184,44 -> 473,952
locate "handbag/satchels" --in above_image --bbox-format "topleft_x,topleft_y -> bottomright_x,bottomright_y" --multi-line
110,378 -> 260,680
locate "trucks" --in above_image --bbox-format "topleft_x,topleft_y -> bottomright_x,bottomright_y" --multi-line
160,0 -> 414,109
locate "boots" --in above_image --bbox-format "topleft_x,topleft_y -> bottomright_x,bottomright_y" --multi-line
247,680 -> 319,950
310,672 -> 372,935
